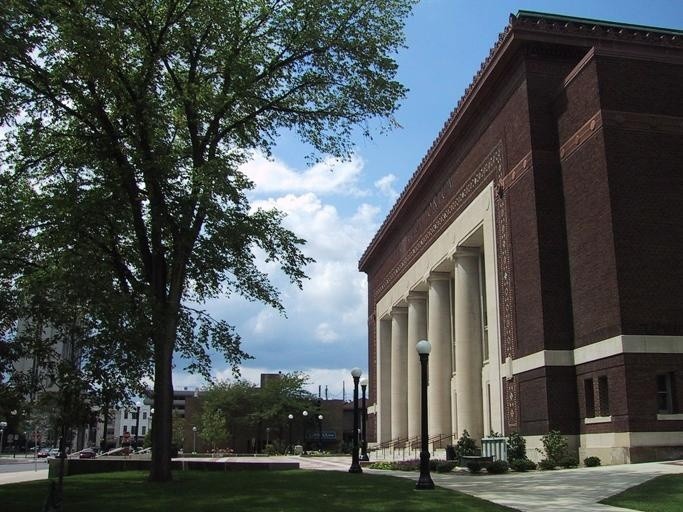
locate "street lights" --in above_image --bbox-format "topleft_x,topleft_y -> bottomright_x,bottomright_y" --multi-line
357,428 -> 361,458
133,400 -> 140,453
265,426 -> 271,453
415,340 -> 434,489
359,377 -> 369,461
302,410 -> 308,455
318,414 -> 323,452
288,415 -> 292,454
191,426 -> 197,454
349,366 -> 362,475
150,408 -> 153,455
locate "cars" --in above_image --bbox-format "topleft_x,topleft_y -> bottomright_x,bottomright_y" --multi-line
35,448 -> 50,458
29,446 -> 183,455
79,448 -> 96,458
46,448 -> 67,459
